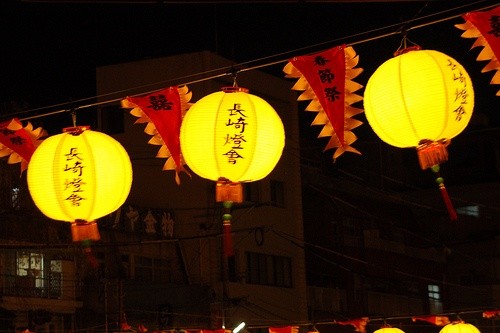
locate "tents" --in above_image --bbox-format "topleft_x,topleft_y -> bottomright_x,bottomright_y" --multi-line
179,73 -> 285,255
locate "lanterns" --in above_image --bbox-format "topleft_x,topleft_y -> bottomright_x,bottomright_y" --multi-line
363,29 -> 476,221
26,111 -> 133,269
438,313 -> 481,333
375,319 -> 405,333
